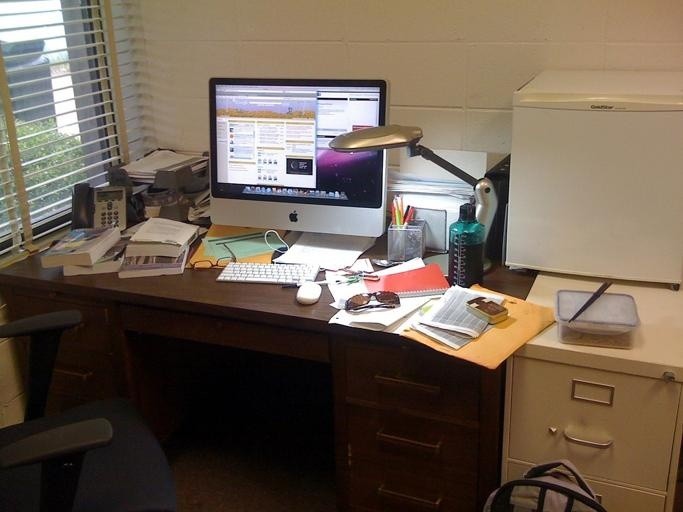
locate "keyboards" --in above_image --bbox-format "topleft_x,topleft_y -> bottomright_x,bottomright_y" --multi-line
216,262 -> 320,285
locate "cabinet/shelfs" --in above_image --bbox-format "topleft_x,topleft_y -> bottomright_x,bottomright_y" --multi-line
484,273 -> 683,511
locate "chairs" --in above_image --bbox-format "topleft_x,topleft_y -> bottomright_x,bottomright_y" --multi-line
1,305 -> 175,512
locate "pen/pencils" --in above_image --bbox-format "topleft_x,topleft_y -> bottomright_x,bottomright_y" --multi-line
391,194 -> 414,259
281,280 -> 340,288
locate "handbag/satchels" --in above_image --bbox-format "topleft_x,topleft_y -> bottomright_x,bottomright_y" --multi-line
484,459 -> 607,512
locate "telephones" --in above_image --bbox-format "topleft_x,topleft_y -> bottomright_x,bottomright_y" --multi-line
71,183 -> 126,231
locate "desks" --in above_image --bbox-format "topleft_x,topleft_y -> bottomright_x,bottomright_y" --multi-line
1,219 -> 539,512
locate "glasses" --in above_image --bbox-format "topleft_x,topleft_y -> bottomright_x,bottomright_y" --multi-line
346,291 -> 400,310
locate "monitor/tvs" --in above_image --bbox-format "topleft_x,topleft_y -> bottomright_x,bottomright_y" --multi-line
209,78 -> 391,247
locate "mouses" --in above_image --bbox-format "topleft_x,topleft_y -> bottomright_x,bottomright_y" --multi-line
296,282 -> 322,304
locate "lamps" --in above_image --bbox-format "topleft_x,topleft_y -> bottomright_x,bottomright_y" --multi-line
329,124 -> 498,280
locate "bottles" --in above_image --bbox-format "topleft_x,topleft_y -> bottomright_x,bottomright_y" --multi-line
448,202 -> 486,288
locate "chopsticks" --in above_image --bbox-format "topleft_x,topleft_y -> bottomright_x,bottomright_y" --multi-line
568,281 -> 614,324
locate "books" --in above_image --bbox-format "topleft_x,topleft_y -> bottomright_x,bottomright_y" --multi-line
63,237 -> 129,276
126,217 -> 200,257
118,247 -> 189,279
363,263 -> 451,297
41,228 -> 121,269
